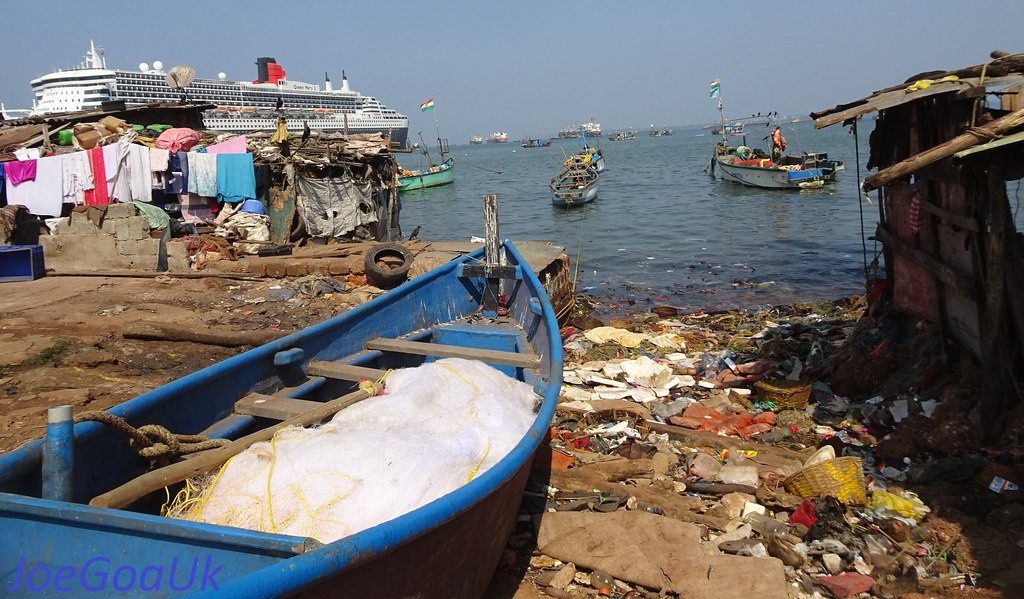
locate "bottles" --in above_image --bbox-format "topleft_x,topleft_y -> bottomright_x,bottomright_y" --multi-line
718,351 -> 737,373
757,426 -> 798,445
588,435 -> 602,455
591,570 -> 626,595
743,510 -> 802,545
709,354 -> 723,369
637,502 -> 672,519
873,533 -> 896,557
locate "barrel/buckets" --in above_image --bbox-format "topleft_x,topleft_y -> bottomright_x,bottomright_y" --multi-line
240,199 -> 264,214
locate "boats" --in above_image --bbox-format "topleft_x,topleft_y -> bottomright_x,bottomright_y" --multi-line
0,239 -> 563,599
708,75 -> 837,190
470,135 -> 482,144
561,123 -> 605,173
660,124 -> 676,135
711,98 -> 744,136
394,98 -> 455,192
731,130 -> 750,136
549,161 -> 599,207
549,117 -> 639,141
648,123 -> 662,136
519,136 -> 552,147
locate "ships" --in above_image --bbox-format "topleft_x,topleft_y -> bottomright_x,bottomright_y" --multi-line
1,39 -> 410,153
486,130 -> 507,143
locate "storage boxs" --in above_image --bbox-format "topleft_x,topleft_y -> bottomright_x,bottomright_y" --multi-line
0,244 -> 46,283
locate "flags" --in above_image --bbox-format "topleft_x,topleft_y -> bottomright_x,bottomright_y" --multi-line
420,97 -> 435,113
709,78 -> 720,99
